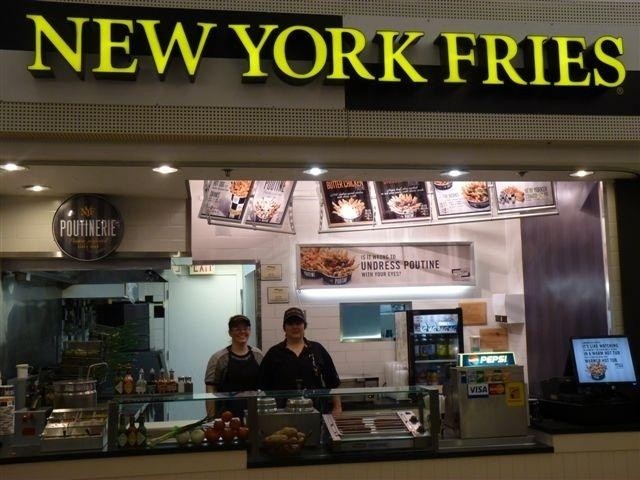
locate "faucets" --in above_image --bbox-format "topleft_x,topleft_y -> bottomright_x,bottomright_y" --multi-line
86,362 -> 108,380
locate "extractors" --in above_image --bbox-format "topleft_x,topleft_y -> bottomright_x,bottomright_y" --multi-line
61,272 -> 140,302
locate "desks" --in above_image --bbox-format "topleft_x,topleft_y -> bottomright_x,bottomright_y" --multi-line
529,425 -> 640,480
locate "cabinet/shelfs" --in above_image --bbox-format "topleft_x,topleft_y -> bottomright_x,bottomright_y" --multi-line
408,311 -> 459,391
1,418 -> 555,480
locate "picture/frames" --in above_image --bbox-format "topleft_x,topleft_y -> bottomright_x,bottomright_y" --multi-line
199,180 -> 254,224
433,180 -> 492,219
261,264 -> 282,281
267,287 -> 289,303
319,181 -> 376,227
245,180 -> 298,225
373,181 -> 433,224
495,182 -> 556,214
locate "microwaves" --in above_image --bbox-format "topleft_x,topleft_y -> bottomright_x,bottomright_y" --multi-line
337,375 -> 380,405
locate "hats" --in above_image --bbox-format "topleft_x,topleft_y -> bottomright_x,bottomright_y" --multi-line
229,315 -> 251,329
283,308 -> 307,332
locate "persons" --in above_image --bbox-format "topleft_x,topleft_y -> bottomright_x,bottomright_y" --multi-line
204,315 -> 264,421
260,308 -> 342,414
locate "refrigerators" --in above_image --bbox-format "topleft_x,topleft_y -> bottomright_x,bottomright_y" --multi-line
395,309 -> 464,403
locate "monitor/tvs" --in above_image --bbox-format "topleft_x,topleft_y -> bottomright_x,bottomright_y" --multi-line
569,333 -> 638,386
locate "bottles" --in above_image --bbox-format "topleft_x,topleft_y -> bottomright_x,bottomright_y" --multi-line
159,369 -> 168,394
118,414 -> 127,448
448,335 -> 456,360
437,333 -> 447,360
420,334 -> 429,360
137,413 -> 148,448
147,368 -> 159,395
414,334 -> 420,361
186,377 -> 193,394
123,363 -> 134,393
136,368 -> 146,395
127,413 -> 137,448
429,334 -> 436,360
179,376 -> 185,393
168,369 -> 178,393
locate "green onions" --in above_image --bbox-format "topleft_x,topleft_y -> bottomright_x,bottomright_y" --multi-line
148,409 -> 215,447
